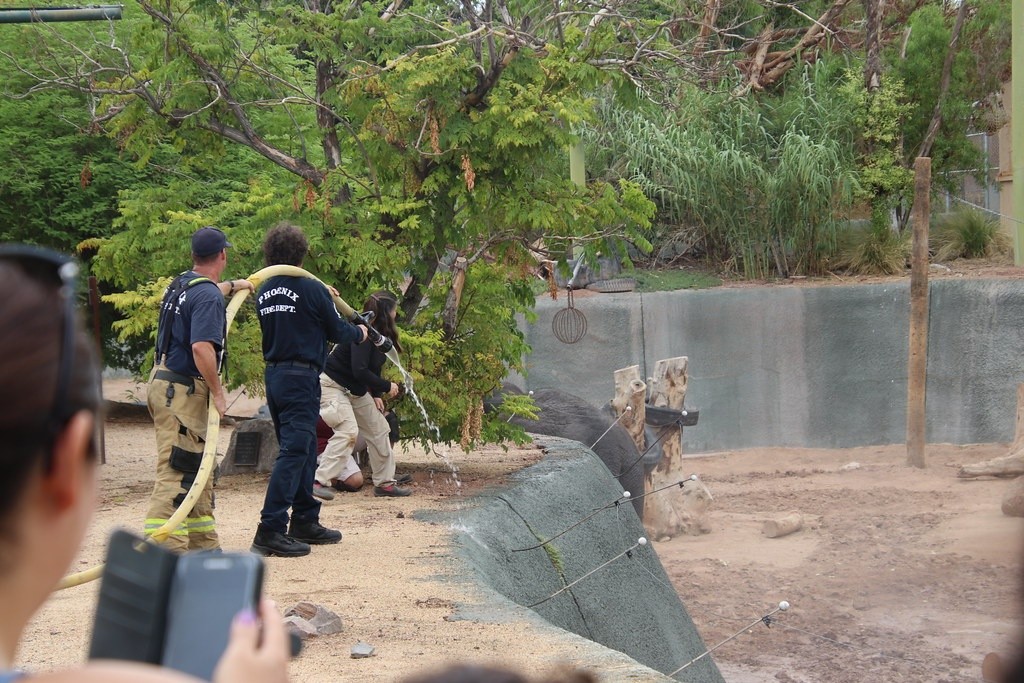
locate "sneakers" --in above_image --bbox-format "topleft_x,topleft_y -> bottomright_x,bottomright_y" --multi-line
251,524 -> 311,557
374,483 -> 411,496
290,519 -> 342,544
311,483 -> 335,501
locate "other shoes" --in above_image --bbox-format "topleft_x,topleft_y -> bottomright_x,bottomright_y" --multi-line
395,473 -> 412,483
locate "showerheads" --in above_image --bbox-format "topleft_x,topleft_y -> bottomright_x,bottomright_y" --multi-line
348,311 -> 393,354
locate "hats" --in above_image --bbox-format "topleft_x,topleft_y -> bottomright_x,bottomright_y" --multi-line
192,227 -> 232,255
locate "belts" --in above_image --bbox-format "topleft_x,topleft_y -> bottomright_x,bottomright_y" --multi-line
153,370 -> 194,388
267,360 -> 316,369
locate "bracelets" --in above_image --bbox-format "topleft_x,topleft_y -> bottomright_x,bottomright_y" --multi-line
229,281 -> 235,296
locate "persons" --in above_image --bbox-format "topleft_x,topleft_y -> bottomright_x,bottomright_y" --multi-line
311,290 -> 412,501
250,221 -> 367,557
144,228 -> 254,554
0,246 -> 288,683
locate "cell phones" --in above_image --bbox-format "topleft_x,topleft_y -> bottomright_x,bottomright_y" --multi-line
162,552 -> 266,680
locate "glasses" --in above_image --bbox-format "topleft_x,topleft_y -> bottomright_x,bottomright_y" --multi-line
6,246 -> 79,470
